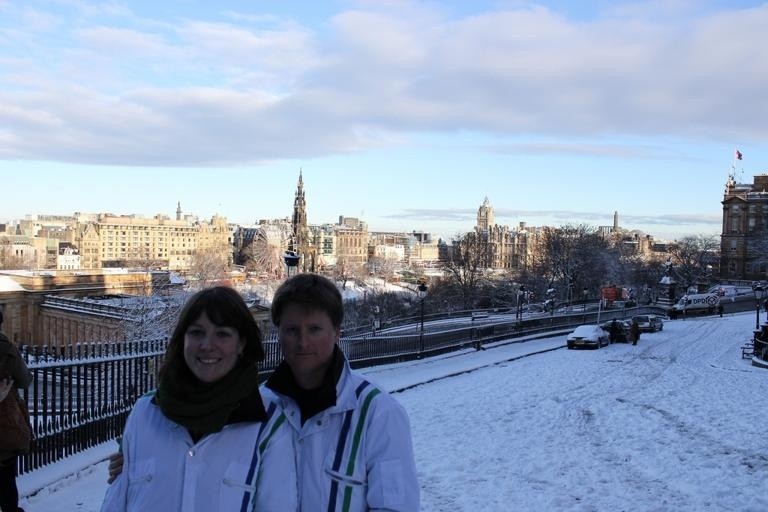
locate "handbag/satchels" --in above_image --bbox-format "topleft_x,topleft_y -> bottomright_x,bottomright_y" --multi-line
0,378 -> 34,454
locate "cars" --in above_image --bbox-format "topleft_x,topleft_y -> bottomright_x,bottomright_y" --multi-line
565,319 -> 641,349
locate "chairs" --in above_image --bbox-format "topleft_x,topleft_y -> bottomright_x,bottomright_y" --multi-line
741,339 -> 755,359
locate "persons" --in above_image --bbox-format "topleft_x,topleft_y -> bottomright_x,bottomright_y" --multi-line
610,317 -> 619,344
108,274 -> 420,512
0,311 -> 33,512
101,286 -> 297,512
717,304 -> 724,316
629,319 -> 638,345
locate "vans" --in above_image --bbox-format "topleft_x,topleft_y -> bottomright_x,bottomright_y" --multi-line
631,313 -> 664,333
671,293 -> 722,315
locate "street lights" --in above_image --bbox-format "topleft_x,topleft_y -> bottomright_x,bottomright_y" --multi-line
416,279 -> 430,351
751,281 -> 766,329
516,285 -> 526,338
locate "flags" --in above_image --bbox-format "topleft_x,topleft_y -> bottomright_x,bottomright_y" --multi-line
736,150 -> 742,160
